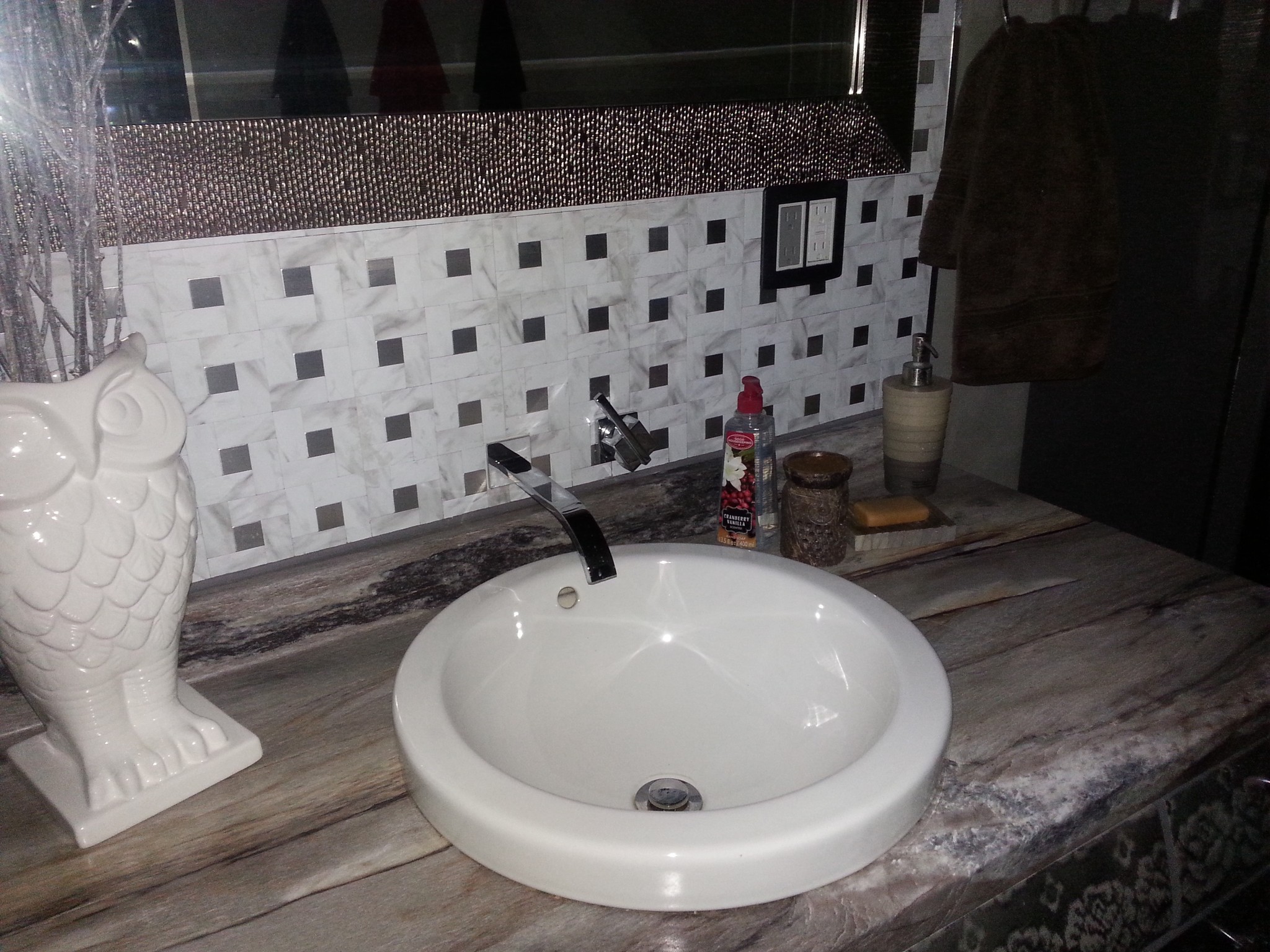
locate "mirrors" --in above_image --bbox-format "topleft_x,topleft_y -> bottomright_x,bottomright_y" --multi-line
0,0 -> 926,266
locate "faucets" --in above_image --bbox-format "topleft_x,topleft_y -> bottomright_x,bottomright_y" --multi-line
483,437 -> 622,589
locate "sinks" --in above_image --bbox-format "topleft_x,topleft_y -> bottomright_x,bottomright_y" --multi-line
388,542 -> 955,917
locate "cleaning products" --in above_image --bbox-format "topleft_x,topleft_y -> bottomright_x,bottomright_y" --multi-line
714,373 -> 780,550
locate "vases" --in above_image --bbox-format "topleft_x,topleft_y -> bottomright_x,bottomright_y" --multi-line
0,332 -> 267,855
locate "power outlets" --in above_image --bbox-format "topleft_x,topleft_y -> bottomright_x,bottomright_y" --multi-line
758,178 -> 849,291
